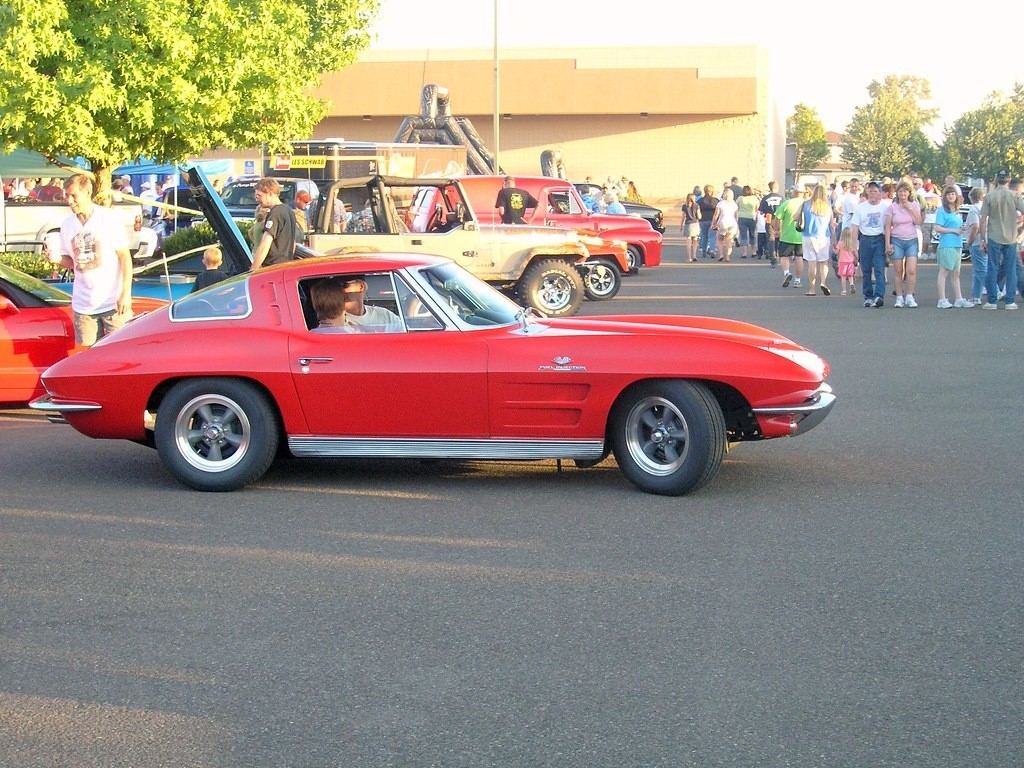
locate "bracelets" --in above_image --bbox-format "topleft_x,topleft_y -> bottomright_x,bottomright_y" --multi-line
981,239 -> 986,242
909,207 -> 912,210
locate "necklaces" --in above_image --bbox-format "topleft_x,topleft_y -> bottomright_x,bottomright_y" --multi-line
318,321 -> 336,327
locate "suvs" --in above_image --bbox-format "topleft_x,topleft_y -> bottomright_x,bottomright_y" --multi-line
305,176 -> 589,318
405,177 -> 663,276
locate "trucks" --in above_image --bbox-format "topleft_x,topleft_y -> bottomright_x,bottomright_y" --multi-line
259,141 -> 466,182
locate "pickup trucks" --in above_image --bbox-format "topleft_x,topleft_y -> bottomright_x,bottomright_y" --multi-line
0,199 -> 142,269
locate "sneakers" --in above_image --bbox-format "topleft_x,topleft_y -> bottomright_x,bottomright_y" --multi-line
936,285 -> 1019,310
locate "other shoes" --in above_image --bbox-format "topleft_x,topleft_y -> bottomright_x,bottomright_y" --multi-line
782,273 -> 792,287
841,283 -> 856,295
687,257 -> 698,263
863,297 -> 884,308
793,277 -> 802,288
805,291 -> 816,296
698,246 -> 730,262
734,237 -> 740,247
892,290 -> 918,308
741,254 -> 777,268
920,252 -> 937,260
820,285 -> 830,296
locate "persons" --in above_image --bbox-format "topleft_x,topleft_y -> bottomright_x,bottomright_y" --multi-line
248,177 -> 376,271
46,174 -> 133,346
680,171 -> 1024,310
579,175 -> 645,215
190,247 -> 230,292
495,176 -> 541,224
309,271 -> 402,333
3,175 -> 221,252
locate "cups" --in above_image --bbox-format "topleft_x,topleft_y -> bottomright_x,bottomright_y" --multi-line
45,232 -> 62,264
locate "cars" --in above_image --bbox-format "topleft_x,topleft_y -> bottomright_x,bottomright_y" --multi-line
140,185 -> 204,248
190,177 -> 318,225
28,252 -> 837,496
571,183 -> 665,236
0,263 -> 174,402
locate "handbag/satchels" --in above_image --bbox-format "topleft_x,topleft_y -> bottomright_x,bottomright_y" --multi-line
712,225 -> 719,230
795,201 -> 805,232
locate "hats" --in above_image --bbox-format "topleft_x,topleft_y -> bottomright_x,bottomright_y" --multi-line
505,176 -> 514,181
112,179 -> 123,185
868,182 -> 881,188
121,175 -> 130,181
924,183 -> 934,190
140,182 -> 150,188
297,190 -> 312,202
731,177 -> 738,183
621,177 -> 629,181
997,169 -> 1010,177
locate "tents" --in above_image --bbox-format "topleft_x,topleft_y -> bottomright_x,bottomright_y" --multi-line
0,144 -> 91,194
68,152 -> 235,233
